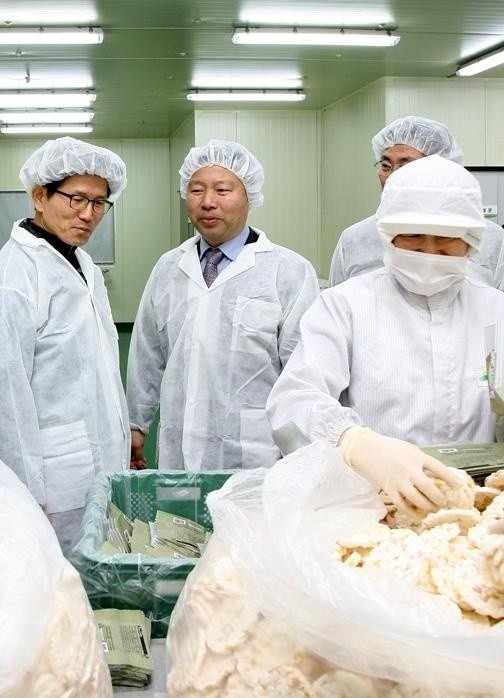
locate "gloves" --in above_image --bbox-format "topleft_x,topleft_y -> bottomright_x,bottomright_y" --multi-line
339,425 -> 467,526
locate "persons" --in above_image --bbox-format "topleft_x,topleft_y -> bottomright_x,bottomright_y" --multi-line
330,115 -> 503,289
124,142 -> 321,472
263,153 -> 504,524
1,136 -> 133,564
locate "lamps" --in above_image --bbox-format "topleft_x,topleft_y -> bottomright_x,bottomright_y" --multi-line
233,27 -> 400,44
1,89 -> 96,108
0,26 -> 103,46
187,88 -> 306,102
1,125 -> 93,132
0,108 -> 93,122
456,49 -> 504,77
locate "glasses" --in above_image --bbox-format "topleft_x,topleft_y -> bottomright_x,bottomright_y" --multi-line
373,157 -> 408,176
42,183 -> 115,217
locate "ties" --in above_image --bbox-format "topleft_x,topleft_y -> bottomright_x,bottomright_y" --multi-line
202,249 -> 226,290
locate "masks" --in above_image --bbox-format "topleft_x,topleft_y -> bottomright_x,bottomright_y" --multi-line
383,246 -> 470,296
